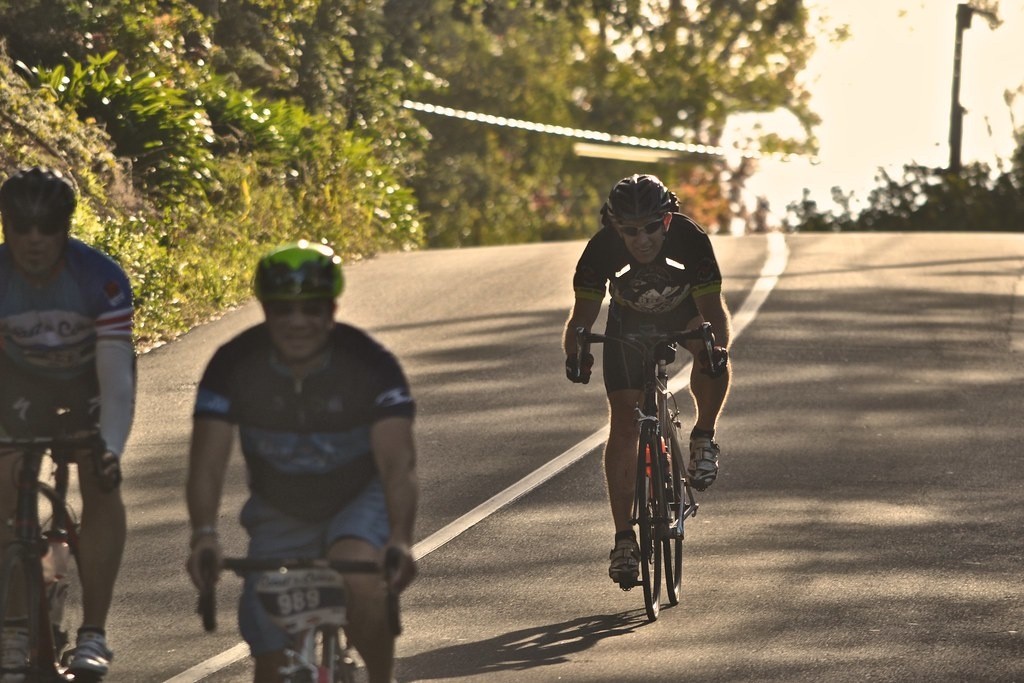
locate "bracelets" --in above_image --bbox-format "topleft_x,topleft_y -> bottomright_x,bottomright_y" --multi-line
190,528 -> 217,548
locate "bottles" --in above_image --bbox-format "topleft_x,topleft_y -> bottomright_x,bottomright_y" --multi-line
645,428 -> 667,476
39,535 -> 55,586
648,445 -> 672,502
40,529 -> 70,624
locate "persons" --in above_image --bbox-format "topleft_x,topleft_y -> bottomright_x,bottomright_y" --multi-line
187,243 -> 419,683
564,175 -> 729,582
0,166 -> 137,683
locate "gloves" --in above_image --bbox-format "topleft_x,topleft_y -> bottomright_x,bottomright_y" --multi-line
697,345 -> 728,378
565,353 -> 594,384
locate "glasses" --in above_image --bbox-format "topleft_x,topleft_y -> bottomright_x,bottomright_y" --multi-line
616,214 -> 667,238
269,300 -> 334,316
9,220 -> 65,236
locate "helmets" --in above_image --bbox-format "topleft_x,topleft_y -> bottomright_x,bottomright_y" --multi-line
607,174 -> 671,220
254,248 -> 343,300
0,163 -> 77,222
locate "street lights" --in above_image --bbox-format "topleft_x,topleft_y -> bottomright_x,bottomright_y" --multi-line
949,2 -> 998,224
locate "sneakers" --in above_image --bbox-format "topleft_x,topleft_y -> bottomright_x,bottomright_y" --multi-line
69,627 -> 113,675
609,535 -> 640,583
688,426 -> 720,488
0,616 -> 30,672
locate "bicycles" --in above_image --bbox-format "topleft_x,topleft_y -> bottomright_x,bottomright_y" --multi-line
571,323 -> 724,618
190,545 -> 408,683
0,424 -> 115,682
